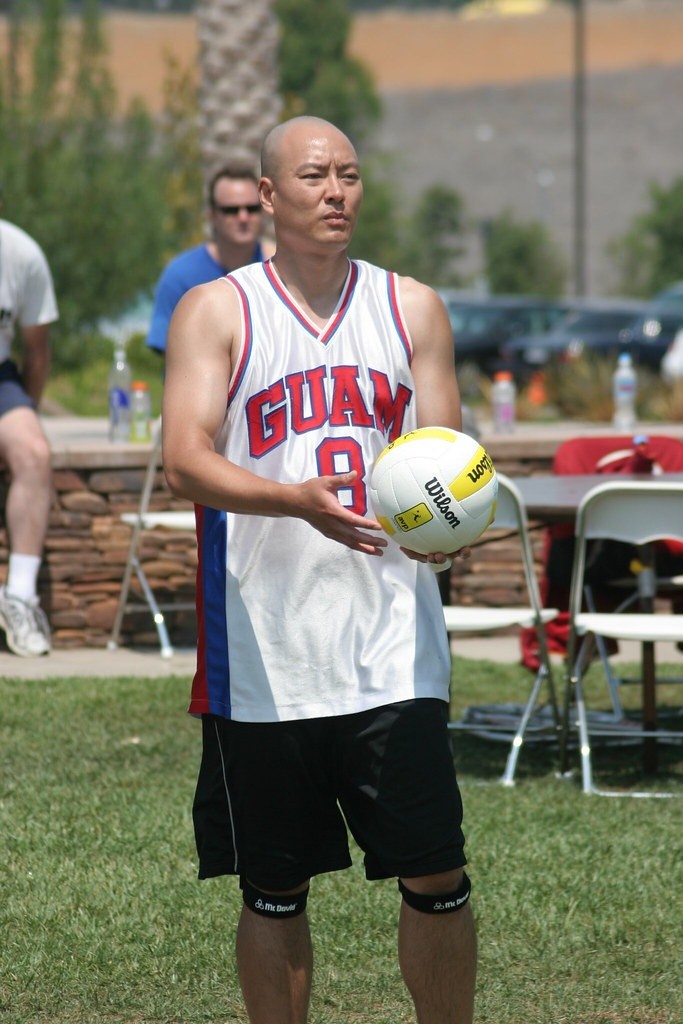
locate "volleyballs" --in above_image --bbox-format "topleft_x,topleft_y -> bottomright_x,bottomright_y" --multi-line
369,426 -> 500,557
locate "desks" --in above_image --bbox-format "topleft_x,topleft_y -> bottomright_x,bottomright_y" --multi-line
503,473 -> 682,759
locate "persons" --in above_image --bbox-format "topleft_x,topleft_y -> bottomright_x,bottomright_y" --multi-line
147,163 -> 273,354
0,216 -> 60,659
159,115 -> 479,1024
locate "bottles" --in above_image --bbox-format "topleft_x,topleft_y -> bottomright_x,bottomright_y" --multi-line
492,371 -> 518,432
129,379 -> 152,446
612,354 -> 639,433
107,349 -> 133,444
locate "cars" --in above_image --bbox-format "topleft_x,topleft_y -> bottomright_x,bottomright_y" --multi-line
432,280 -> 683,401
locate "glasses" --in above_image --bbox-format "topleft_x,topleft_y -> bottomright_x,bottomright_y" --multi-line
213,203 -> 262,214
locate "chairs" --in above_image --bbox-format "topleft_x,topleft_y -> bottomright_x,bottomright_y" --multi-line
99,407 -> 198,663
556,479 -> 682,798
481,471 -> 566,793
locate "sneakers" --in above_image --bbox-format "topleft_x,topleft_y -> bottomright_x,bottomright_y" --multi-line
0,586 -> 52,656
